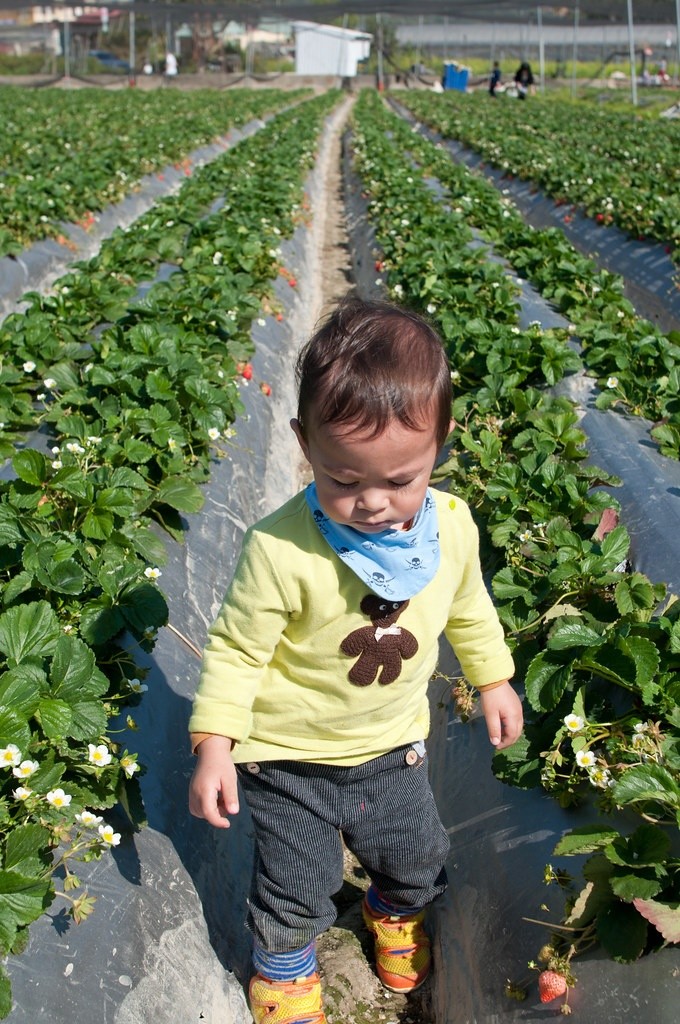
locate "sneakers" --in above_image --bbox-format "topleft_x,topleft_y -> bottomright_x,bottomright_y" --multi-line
363,894 -> 431,993
250,970 -> 329,1024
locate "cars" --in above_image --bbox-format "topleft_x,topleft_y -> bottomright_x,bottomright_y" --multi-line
92,50 -> 130,73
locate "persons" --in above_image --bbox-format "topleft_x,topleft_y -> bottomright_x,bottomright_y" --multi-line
134,37 -> 670,103
187,290 -> 525,1024
